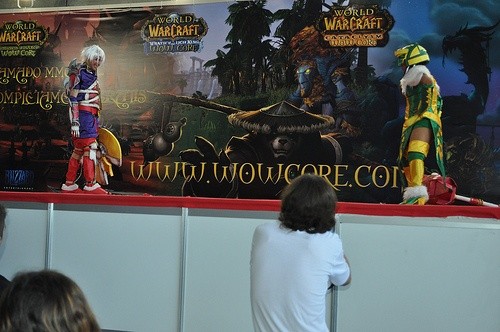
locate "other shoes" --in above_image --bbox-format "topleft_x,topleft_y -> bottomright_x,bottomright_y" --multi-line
62,183 -> 85,193
399,185 -> 429,205
83,183 -> 109,194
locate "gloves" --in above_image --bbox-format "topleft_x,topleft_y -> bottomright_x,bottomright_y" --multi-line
71,122 -> 80,138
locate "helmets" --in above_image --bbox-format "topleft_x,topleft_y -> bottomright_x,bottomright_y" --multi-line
394,43 -> 432,66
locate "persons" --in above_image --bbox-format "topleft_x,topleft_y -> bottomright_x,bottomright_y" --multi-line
394,43 -> 445,204
59,44 -> 113,194
0,270 -> 103,332
249,177 -> 351,332
